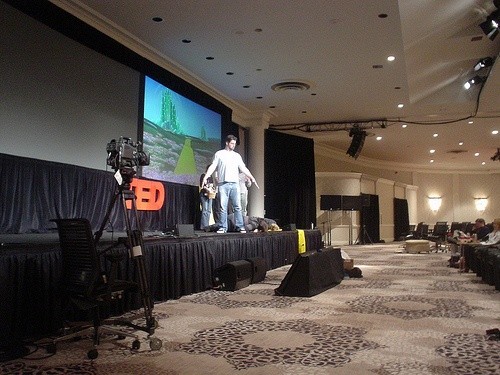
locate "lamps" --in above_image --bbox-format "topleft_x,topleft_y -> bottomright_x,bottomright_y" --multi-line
465,76 -> 483,90
474,56 -> 496,72
478,8 -> 500,41
430,196 -> 442,215
348,127 -> 365,159
474,197 -> 490,215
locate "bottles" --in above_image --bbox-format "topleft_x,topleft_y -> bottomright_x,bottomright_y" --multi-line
311,223 -> 314,230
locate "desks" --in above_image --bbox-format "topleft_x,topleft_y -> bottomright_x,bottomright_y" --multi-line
448,236 -> 481,274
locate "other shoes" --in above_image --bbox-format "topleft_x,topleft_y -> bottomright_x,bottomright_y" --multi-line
217,227 -> 228,234
239,228 -> 246,234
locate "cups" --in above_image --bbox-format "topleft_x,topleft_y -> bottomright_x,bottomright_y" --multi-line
473,233 -> 477,240
467,233 -> 470,239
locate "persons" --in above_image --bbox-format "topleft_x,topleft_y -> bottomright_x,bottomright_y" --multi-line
213,168 -> 251,231
454,219 -> 500,273
448,219 -> 489,267
199,164 -> 215,231
201,135 -> 259,234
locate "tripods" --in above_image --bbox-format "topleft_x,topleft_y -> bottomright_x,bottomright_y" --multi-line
91,179 -> 155,328
353,206 -> 373,245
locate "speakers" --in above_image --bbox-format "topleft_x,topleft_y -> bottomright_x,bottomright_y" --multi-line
360,194 -> 371,207
243,256 -> 266,283
211,260 -> 253,292
171,223 -> 194,237
346,131 -> 366,160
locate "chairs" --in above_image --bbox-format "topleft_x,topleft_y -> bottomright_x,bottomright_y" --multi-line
46,217 -> 141,359
407,223 -> 500,291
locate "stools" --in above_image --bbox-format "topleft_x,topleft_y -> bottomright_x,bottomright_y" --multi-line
405,239 -> 431,254
